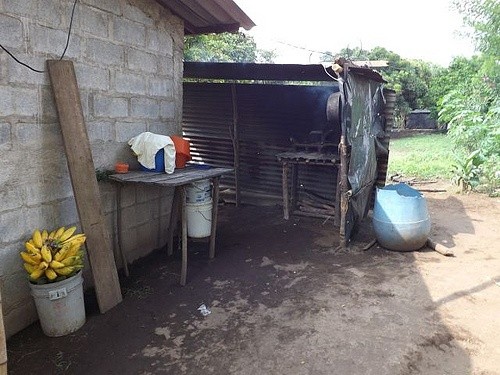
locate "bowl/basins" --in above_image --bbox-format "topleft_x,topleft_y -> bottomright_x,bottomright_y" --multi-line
114,163 -> 128,172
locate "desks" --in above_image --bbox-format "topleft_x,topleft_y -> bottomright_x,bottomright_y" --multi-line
108,164 -> 235,286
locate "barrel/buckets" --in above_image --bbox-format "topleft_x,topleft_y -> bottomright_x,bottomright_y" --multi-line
27,270 -> 87,338
183,177 -> 213,240
140,143 -> 164,172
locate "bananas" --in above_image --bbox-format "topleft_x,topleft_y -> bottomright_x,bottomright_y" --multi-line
18,225 -> 87,283
